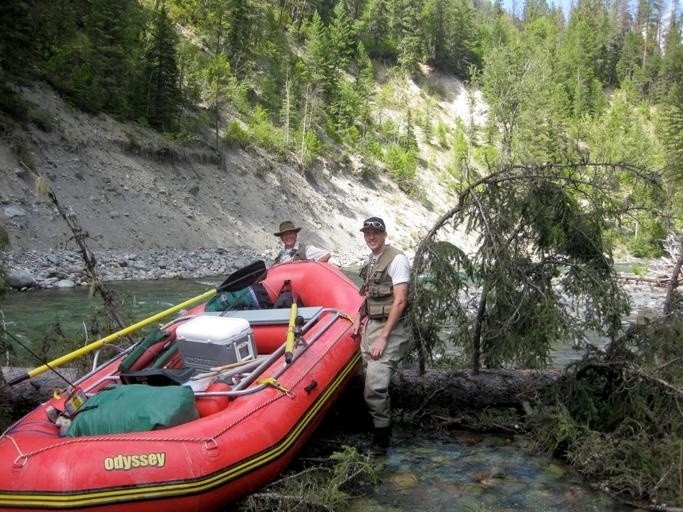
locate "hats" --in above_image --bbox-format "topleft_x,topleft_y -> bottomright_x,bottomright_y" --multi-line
360,217 -> 385,232
273,221 -> 302,237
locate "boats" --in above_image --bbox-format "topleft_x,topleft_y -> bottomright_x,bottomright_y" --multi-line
0,260 -> 369,512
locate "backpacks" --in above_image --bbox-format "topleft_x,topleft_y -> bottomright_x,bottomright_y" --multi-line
205,282 -> 273,312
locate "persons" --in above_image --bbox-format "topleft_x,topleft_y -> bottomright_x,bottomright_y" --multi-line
354,216 -> 418,452
270,220 -> 332,265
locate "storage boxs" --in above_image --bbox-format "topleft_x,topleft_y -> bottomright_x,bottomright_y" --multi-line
176,315 -> 257,374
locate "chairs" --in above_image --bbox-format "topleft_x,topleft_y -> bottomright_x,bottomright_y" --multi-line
120,368 -> 197,386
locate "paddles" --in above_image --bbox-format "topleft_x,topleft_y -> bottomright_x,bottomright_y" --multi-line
8,260 -> 266,388
284,291 -> 302,362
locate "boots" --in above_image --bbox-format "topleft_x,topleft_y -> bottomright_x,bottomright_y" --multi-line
369,425 -> 391,456
